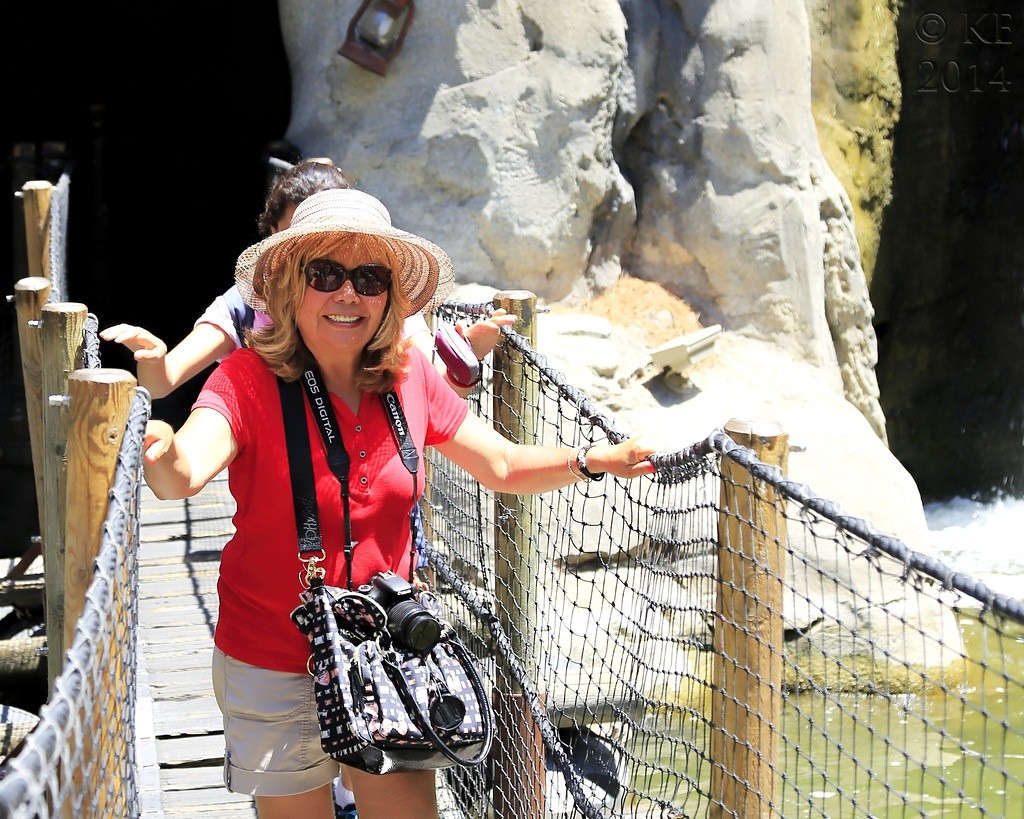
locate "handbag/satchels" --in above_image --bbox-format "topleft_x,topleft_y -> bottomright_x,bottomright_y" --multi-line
290,585 -> 498,775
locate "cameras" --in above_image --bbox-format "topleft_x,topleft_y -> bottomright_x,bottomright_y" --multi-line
356,569 -> 440,655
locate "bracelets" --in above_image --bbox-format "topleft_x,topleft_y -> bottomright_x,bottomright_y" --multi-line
446,369 -> 479,388
569,448 -> 592,482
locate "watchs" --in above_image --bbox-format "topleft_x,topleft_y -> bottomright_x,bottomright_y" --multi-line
577,445 -> 606,481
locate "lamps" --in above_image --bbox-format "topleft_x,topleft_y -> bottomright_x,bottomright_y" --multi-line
337,0 -> 415,77
651,324 -> 726,394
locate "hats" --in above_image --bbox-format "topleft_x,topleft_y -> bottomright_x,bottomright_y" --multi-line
234,189 -> 456,318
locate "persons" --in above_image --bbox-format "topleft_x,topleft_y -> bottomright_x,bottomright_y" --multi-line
100,162 -> 517,819
144,189 -> 667,819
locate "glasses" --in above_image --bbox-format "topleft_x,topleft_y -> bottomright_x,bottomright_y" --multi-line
299,259 -> 393,297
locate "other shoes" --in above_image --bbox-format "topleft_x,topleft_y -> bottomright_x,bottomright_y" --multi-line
331,777 -> 358,819
